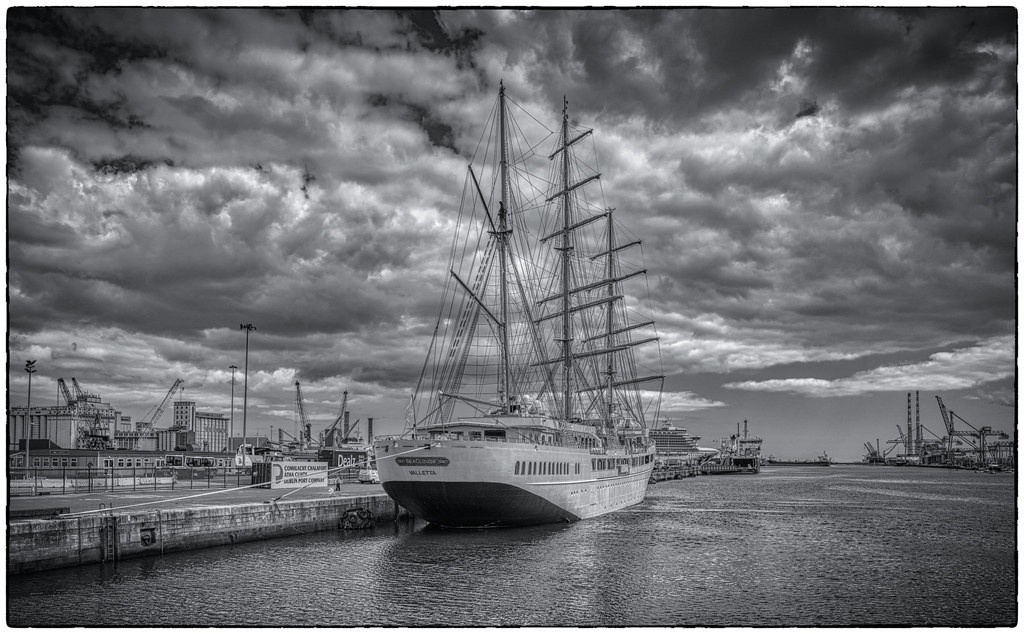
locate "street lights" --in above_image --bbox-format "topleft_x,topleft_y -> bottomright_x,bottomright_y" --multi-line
239,322 -> 257,469
229,364 -> 237,455
25,359 -> 38,478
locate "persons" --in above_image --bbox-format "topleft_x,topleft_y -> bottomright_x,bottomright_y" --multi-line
335,475 -> 341,491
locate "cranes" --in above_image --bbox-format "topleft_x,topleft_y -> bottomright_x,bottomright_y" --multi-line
56,377 -> 185,451
864,389 -> 1009,465
277,382 -> 363,458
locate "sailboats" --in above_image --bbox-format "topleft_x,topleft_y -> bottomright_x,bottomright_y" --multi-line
373,80 -> 668,522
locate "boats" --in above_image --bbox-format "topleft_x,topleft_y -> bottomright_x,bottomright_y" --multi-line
698,419 -> 767,472
651,417 -> 720,467
